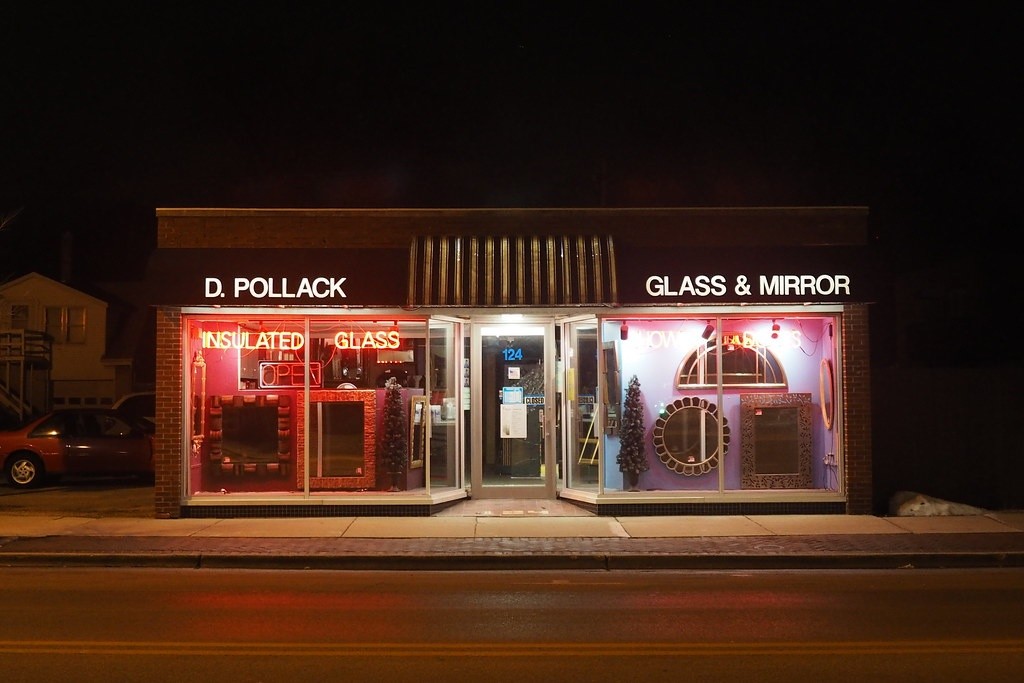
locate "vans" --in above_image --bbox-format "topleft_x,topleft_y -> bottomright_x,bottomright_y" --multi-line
107,393 -> 157,435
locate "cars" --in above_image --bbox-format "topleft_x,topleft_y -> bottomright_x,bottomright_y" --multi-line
0,405 -> 156,489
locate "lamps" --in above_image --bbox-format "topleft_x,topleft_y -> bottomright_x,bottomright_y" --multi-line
702,320 -> 714,339
771,320 -> 780,339
621,321 -> 628,340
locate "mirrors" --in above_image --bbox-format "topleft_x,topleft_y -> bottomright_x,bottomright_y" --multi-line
296,390 -> 375,489
601,340 -> 622,435
410,396 -> 427,468
740,393 -> 814,489
653,396 -> 731,476
211,395 -> 290,478
191,350 -> 206,452
820,358 -> 834,430
